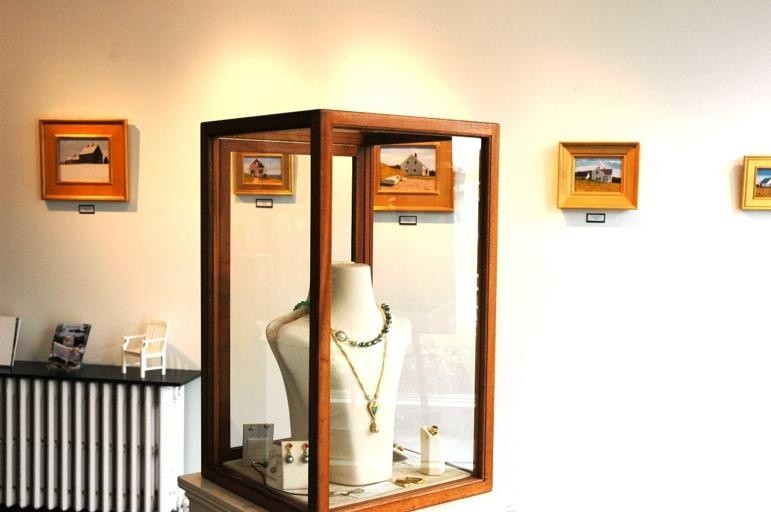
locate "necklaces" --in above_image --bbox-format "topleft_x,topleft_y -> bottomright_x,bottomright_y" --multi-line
293,298 -> 391,346
328,332 -> 388,432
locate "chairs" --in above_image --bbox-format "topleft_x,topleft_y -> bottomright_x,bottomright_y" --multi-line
121,320 -> 169,378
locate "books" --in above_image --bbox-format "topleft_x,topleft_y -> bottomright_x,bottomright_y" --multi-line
51,342 -> 78,363
49,320 -> 91,372
0,316 -> 22,368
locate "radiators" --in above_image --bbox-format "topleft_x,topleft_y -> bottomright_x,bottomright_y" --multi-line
0,377 -> 185,511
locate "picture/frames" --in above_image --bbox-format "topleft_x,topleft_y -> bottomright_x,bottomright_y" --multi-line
232,152 -> 294,196
39,119 -> 128,201
741,155 -> 771,211
558,142 -> 640,210
373,139 -> 453,212
